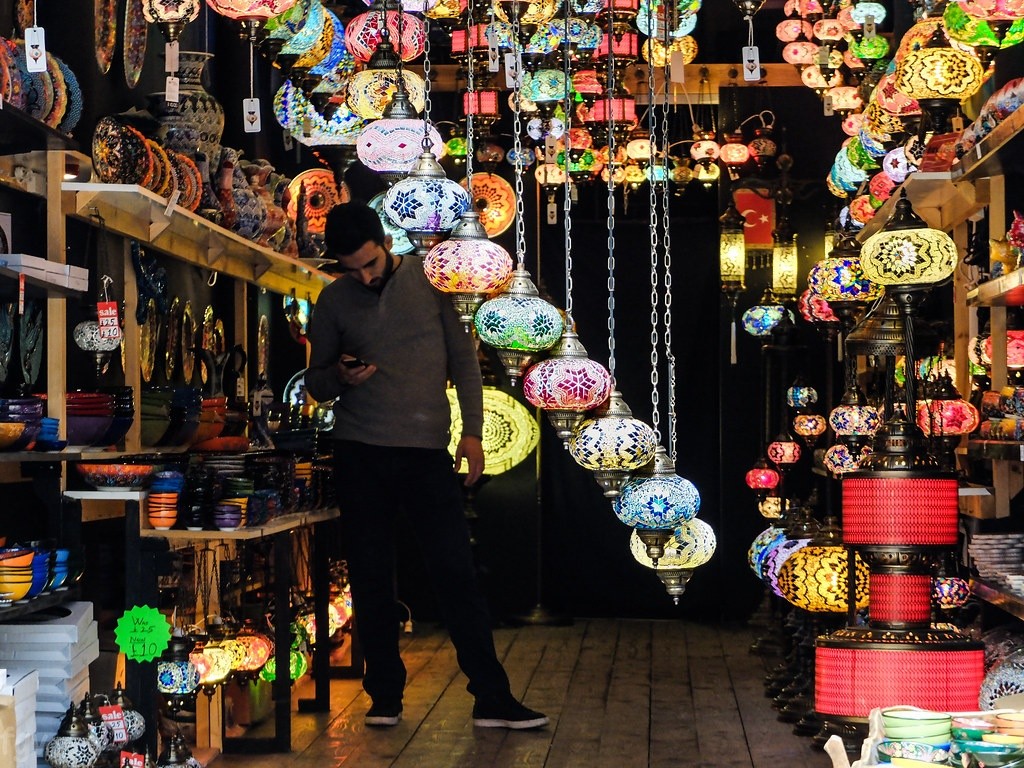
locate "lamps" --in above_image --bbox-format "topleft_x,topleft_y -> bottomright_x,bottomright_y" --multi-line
719,202 -> 748,310
459,173 -> 517,239
770,215 -> 799,309
825,229 -> 835,259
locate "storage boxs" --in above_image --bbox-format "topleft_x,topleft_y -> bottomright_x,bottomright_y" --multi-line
0,602 -> 101,768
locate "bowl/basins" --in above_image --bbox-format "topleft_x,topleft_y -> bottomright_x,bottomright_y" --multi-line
997,727 -> 1024,735
890,734 -> 950,745
147,470 -> 207,531
886,722 -> 952,738
982,735 -> 1024,744
877,741 -> 948,763
206,454 -> 256,529
882,710 -> 951,727
141,384 -> 250,452
952,718 -> 994,728
255,449 -> 312,527
996,713 -> 1024,726
0,394 -> 67,453
951,727 -> 995,741
0,549 -> 70,602
65,384 -> 134,447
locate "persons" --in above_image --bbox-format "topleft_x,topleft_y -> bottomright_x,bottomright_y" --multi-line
304,200 -> 554,730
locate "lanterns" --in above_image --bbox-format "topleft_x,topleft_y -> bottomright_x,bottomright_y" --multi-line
522,322 -> 611,451
715,193 -> 1024,735
473,261 -> 563,387
421,205 -> 513,334
383,146 -> 469,262
43,580 -> 360,768
138,0 -> 1024,193
568,389 -> 657,500
610,444 -> 702,570
72,293 -> 123,379
629,516 -> 716,606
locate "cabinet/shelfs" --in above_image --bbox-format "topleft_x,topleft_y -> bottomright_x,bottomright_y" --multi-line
0,97 -> 364,768
855,102 -> 1024,623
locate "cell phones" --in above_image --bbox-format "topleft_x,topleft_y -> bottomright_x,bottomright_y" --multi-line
341,358 -> 370,369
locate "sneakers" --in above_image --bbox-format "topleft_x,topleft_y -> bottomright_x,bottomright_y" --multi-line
365,696 -> 403,725
473,693 -> 550,729
